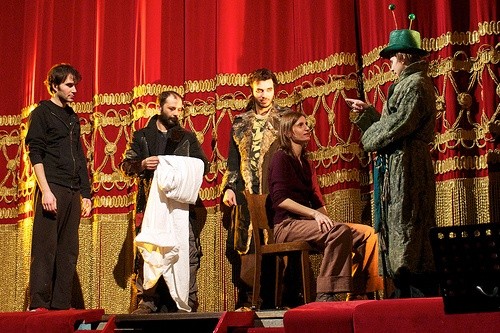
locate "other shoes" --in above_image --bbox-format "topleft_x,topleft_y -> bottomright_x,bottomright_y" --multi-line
316,292 -> 339,302
132,305 -> 150,314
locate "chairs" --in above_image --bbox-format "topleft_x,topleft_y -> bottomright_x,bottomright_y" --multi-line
244,188 -> 323,312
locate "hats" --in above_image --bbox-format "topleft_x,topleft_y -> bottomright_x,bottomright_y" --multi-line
378,5 -> 429,57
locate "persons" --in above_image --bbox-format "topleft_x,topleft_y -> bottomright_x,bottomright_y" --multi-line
117,90 -> 211,317
343,4 -> 439,304
218,68 -> 294,314
24,62 -> 96,313
260,110 -> 383,304
